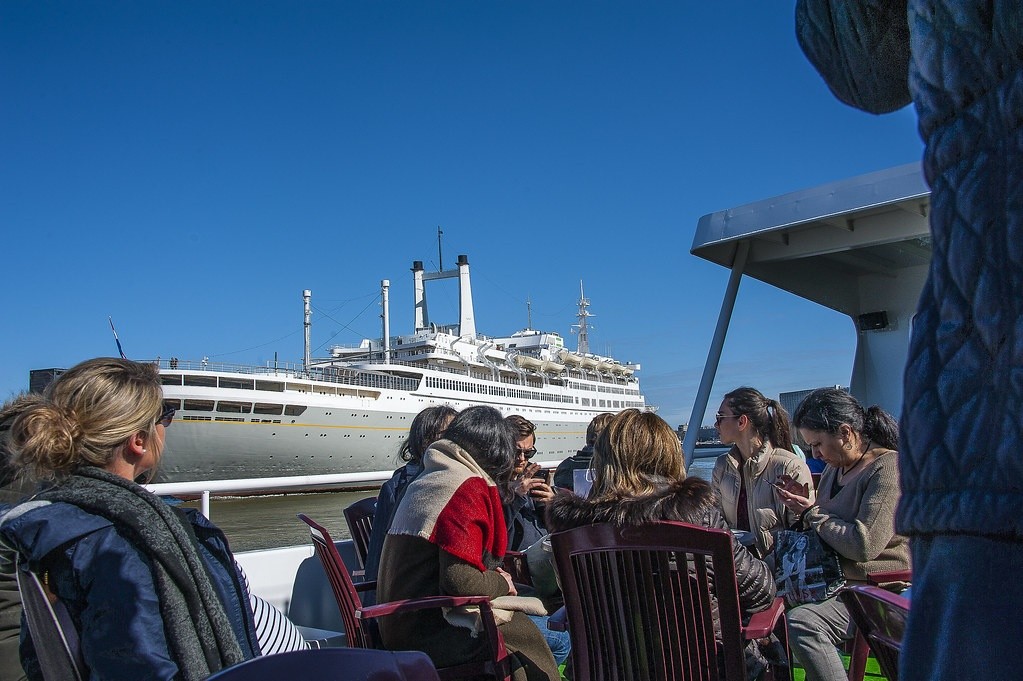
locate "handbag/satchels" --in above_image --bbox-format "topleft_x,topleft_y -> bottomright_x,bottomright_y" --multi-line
775,506 -> 845,605
508,536 -> 563,599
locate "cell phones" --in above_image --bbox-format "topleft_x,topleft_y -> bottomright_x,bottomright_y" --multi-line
527,469 -> 549,498
763,478 -> 782,491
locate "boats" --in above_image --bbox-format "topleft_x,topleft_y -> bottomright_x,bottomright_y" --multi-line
580,355 -> 599,370
612,362 -> 625,374
513,355 -> 544,370
623,368 -> 633,376
545,361 -> 565,375
29,252 -> 660,499
596,360 -> 614,373
553,349 -> 583,365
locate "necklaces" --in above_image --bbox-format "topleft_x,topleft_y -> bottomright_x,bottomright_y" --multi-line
839,441 -> 872,482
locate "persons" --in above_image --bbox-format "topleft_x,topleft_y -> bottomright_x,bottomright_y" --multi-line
363,406 -> 571,681
794,1 -> 1023,680
547,409 -> 778,681
776,388 -> 910,681
554,413 -> 616,492
1,358 -> 314,681
712,387 -> 816,561
170,357 -> 178,370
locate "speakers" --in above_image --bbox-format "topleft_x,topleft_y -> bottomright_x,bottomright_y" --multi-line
857,311 -> 888,330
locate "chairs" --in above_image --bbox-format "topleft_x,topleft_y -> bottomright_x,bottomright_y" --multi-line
343,495 -> 526,583
838,583 -> 912,681
298,512 -> 511,681
551,519 -> 793,681
845,566 -> 913,681
14,555 -> 439,681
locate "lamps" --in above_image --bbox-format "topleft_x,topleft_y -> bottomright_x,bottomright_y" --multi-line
854,308 -> 897,336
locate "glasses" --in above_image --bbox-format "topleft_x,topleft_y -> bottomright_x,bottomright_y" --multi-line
156,404 -> 176,427
716,414 -> 752,426
513,446 -> 537,459
